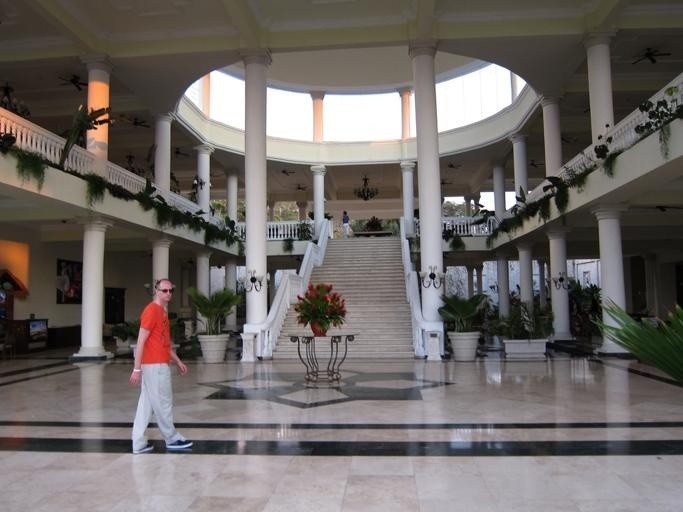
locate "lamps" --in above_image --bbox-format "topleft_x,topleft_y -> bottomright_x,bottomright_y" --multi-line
418,266 -> 446,289
353,175 -> 379,202
237,269 -> 264,292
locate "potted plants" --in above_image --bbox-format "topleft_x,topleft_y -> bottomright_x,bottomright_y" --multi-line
436,290 -> 556,363
109,284 -> 241,362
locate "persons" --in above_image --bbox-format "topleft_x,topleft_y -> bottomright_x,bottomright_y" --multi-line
343,211 -> 349,236
129,278 -> 194,455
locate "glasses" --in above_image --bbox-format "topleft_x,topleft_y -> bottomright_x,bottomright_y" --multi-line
157,289 -> 172,293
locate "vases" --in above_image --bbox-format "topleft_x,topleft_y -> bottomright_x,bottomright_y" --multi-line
310,319 -> 327,336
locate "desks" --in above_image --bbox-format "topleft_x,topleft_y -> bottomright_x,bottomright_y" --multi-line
289,330 -> 359,381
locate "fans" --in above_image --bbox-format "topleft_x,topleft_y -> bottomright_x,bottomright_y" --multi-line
128,117 -> 151,130
630,48 -> 671,65
58,75 -> 88,91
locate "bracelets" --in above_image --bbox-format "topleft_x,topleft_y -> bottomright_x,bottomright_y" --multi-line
133,369 -> 141,372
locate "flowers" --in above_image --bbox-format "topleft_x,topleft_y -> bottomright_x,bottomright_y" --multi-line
296,280 -> 347,330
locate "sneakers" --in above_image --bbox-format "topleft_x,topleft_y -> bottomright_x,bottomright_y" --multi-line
133,444 -> 153,454
166,440 -> 193,449
